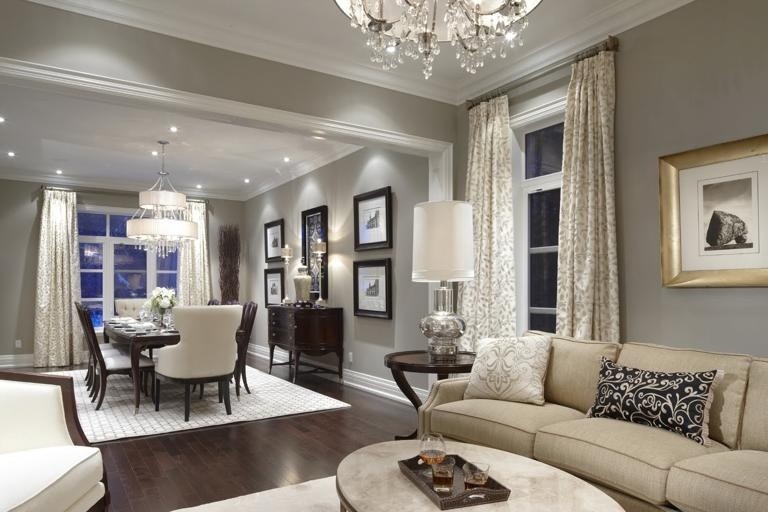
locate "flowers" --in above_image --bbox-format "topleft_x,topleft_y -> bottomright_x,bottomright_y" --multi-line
149,287 -> 178,326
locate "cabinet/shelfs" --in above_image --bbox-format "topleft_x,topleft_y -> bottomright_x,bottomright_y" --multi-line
267,304 -> 344,383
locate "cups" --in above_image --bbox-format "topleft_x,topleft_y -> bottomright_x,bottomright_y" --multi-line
430,456 -> 490,500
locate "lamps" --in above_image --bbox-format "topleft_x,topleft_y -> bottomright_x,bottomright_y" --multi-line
311,238 -> 327,308
280,243 -> 293,306
125,140 -> 199,260
411,200 -> 478,356
333,0 -> 543,82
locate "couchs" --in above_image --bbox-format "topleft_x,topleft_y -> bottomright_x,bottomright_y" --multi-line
415,330 -> 768,511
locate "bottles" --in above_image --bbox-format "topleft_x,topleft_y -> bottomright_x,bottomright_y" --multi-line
294,267 -> 311,301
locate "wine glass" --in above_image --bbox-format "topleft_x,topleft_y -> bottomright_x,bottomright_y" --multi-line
419,432 -> 445,477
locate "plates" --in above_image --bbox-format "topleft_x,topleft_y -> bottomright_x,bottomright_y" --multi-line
110,312 -> 179,335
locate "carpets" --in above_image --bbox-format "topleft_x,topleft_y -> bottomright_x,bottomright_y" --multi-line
169,475 -> 341,512
41,364 -> 353,445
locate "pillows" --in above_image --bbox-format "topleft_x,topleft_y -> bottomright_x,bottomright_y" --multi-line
462,334 -> 554,407
585,354 -> 726,449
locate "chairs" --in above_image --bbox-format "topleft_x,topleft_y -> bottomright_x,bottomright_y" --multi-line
73,297 -> 259,422
0,368 -> 110,512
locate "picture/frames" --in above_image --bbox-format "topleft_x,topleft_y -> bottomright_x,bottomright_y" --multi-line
352,256 -> 394,321
658,132 -> 768,289
263,218 -> 285,263
263,268 -> 285,309
300,205 -> 329,301
352,185 -> 394,252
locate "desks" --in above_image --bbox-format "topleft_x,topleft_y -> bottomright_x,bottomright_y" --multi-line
384,349 -> 477,441
